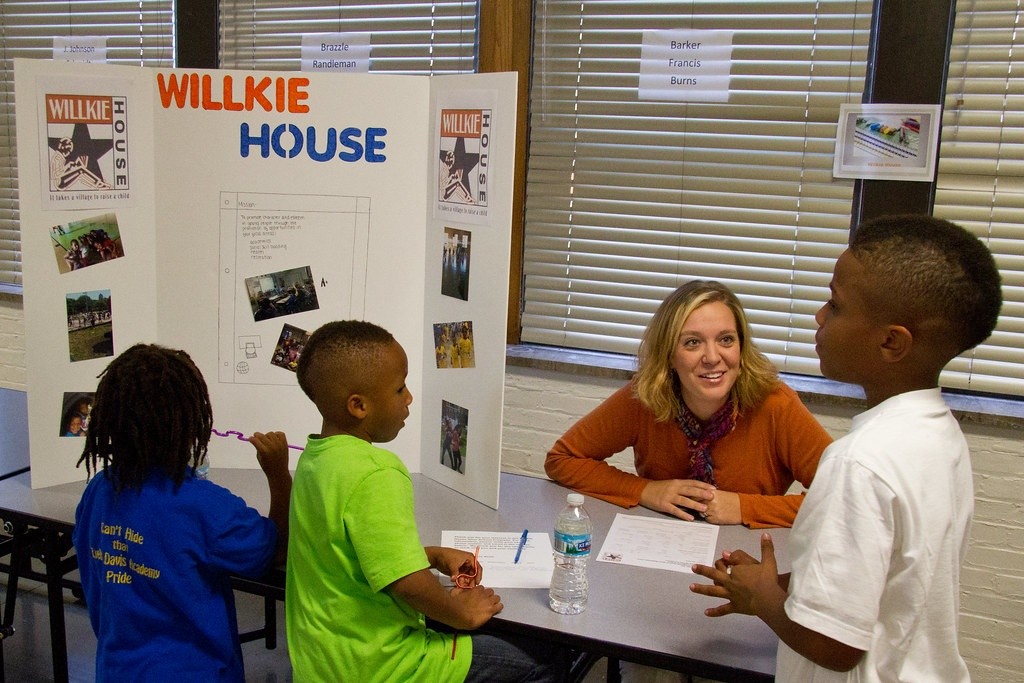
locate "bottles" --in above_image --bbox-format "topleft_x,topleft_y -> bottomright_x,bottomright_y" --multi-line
549,494 -> 592,614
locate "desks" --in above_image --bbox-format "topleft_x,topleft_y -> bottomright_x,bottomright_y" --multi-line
0,472 -> 792,683
1,388 -> 30,634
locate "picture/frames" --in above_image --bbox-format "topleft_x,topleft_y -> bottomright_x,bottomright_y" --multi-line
833,103 -> 941,182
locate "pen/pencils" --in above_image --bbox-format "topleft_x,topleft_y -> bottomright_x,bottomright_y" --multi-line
514,528 -> 529,564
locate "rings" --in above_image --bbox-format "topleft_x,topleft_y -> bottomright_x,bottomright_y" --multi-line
703,511 -> 709,517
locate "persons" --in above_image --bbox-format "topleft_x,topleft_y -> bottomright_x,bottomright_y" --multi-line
441,422 -> 464,475
689,210 -> 1005,683
271,335 -> 311,372
66,397 -> 92,437
254,279 -> 320,322
542,278 -> 834,531
68,310 -> 110,330
62,229 -> 120,271
435,322 -> 473,368
71,344 -> 292,683
285,319 -> 555,683
